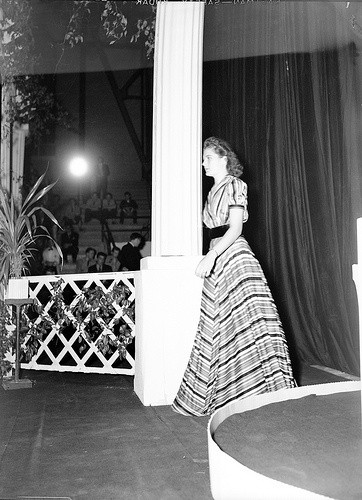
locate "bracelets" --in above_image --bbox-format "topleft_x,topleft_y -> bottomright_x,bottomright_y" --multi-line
210,249 -> 219,258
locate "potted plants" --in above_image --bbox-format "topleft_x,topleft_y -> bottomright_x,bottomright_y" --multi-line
0,161 -> 66,299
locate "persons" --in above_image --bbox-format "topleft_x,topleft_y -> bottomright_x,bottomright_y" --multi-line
119,192 -> 138,224
170,135 -> 298,417
105,246 -> 121,271
41,239 -> 61,275
76,247 -> 97,273
83,192 -> 104,225
94,156 -> 110,201
59,223 -> 79,264
66,197 -> 83,231
46,223 -> 60,248
102,193 -> 118,224
118,232 -> 142,271
88,252 -> 113,273
